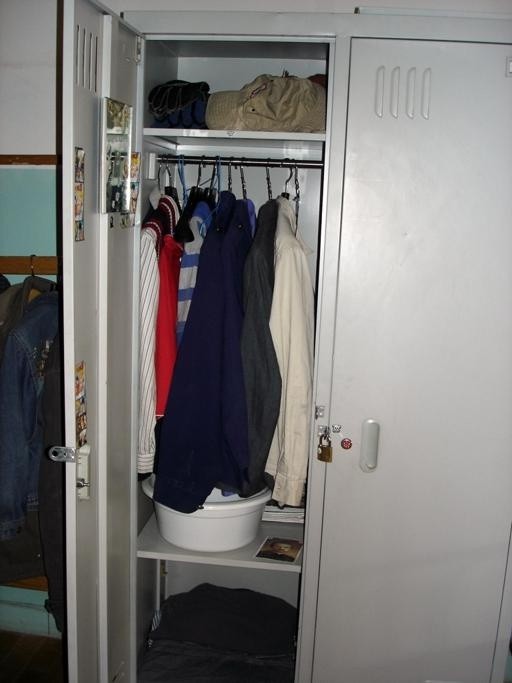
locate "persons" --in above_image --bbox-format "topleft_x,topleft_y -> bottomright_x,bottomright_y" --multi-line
256,540 -> 295,562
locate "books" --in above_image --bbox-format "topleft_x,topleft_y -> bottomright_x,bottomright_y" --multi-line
260,498 -> 306,523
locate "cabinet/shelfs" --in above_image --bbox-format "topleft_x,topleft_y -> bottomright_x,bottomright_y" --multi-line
64,0 -> 512,682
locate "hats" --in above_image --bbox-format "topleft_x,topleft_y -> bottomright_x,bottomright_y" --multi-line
205,74 -> 326,133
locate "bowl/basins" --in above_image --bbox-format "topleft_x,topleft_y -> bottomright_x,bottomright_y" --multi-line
140,476 -> 273,553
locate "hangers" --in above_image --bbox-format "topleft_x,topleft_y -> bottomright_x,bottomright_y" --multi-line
157,154 -> 301,235
23,254 -> 39,298
